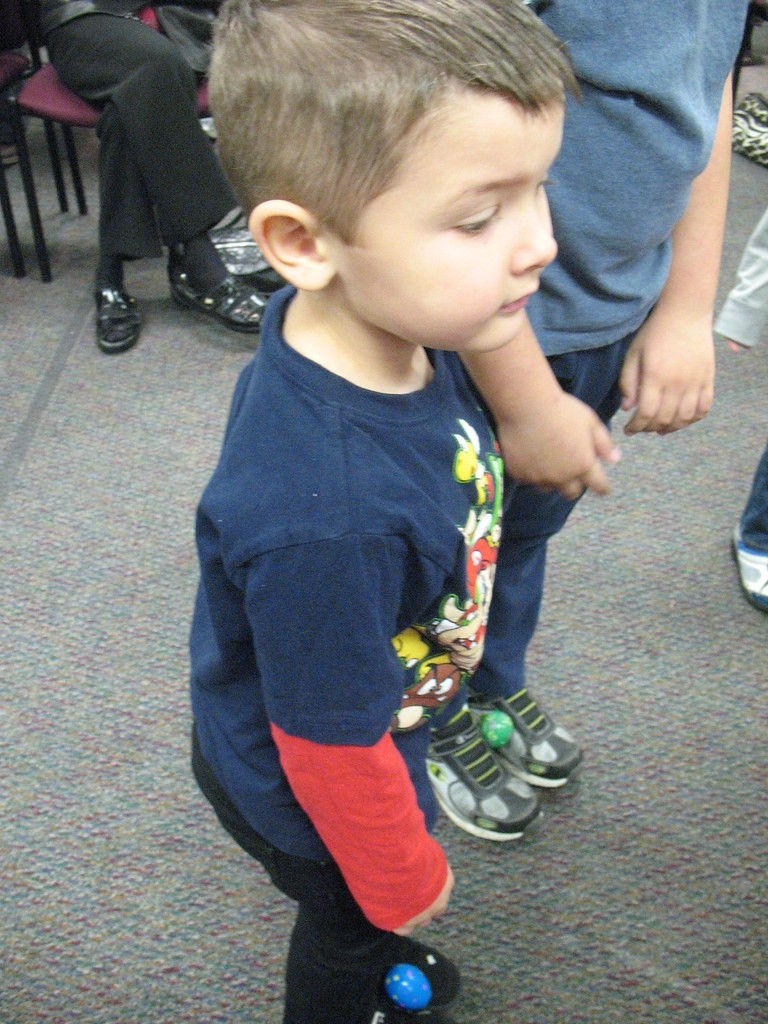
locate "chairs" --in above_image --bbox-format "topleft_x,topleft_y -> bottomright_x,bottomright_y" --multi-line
1,0 -> 214,284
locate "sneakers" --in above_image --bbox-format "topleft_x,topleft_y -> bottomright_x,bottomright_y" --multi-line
424,683 -> 545,842
466,685 -> 585,787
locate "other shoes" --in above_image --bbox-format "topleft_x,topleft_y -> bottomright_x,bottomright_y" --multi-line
732,522 -> 768,612
381,934 -> 460,1009
369,1004 -> 456,1024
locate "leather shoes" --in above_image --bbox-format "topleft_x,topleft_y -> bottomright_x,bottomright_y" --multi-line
168,264 -> 268,334
95,288 -> 141,352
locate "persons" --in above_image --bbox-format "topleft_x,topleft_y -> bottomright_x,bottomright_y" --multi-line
36,0 -> 270,350
191,0 -> 575,1024
424,0 -> 768,842
